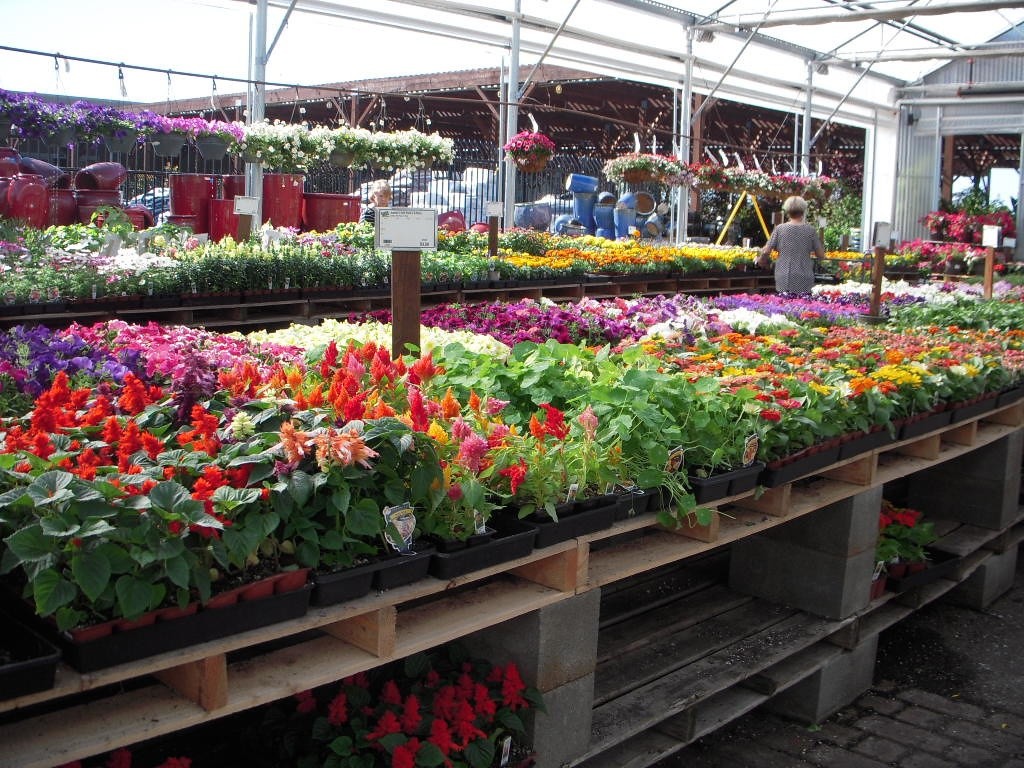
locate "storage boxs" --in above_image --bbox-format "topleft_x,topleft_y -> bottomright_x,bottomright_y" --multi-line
538,497 -> 622,549
999,387 -> 1020,406
0,610 -> 59,699
842,425 -> 903,457
953,398 -> 995,422
890,537 -> 960,591
64,581 -> 314,675
767,445 -> 841,481
900,410 -> 953,440
434,523 -> 540,576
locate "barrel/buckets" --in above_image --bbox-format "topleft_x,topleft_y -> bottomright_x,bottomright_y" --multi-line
208,199 -> 240,244
614,208 -> 636,237
636,212 -> 677,239
555,215 -> 585,239
98,210 -> 144,231
599,191 -> 617,204
617,192 -> 637,209
169,174 -> 217,234
165,215 -> 196,234
566,173 -> 599,193
635,192 -> 657,216
75,157 -> 127,189
0,147 -> 75,227
573,192 -> 597,229
514,202 -> 552,233
592,203 -> 616,229
222,175 -> 246,200
77,190 -> 121,227
470,223 -> 489,234
849,228 -> 861,248
595,229 -> 615,241
263,174 -> 304,237
302,193 -> 362,233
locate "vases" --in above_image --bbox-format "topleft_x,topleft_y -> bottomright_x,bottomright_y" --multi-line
0,176 -> 13,218
314,547 -> 378,607
512,148 -> 548,172
164,214 -> 199,235
688,461 -> 765,506
263,171 -> 303,231
950,263 -> 962,274
378,544 -> 437,592
302,190 -> 362,230
210,196 -> 239,242
222,174 -> 248,197
243,148 -> 263,163
194,135 -> 228,162
21,156 -> 73,189
75,160 -> 128,189
168,173 -> 218,234
75,189 -> 121,221
103,135 -> 136,153
974,262 -> 985,274
693,178 -> 713,188
41,121 -> 74,143
0,146 -> 22,176
930,231 -> 943,240
8,172 -> 48,228
625,170 -> 650,183
329,151 -> 355,170
47,188 -> 76,225
151,134 -> 186,159
0,118 -> 11,144
615,484 -> 674,519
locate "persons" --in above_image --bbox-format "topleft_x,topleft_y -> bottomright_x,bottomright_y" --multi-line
358,179 -> 391,227
757,196 -> 824,292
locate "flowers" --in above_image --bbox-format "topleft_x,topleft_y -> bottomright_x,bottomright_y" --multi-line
0,92 -> 1024,768
